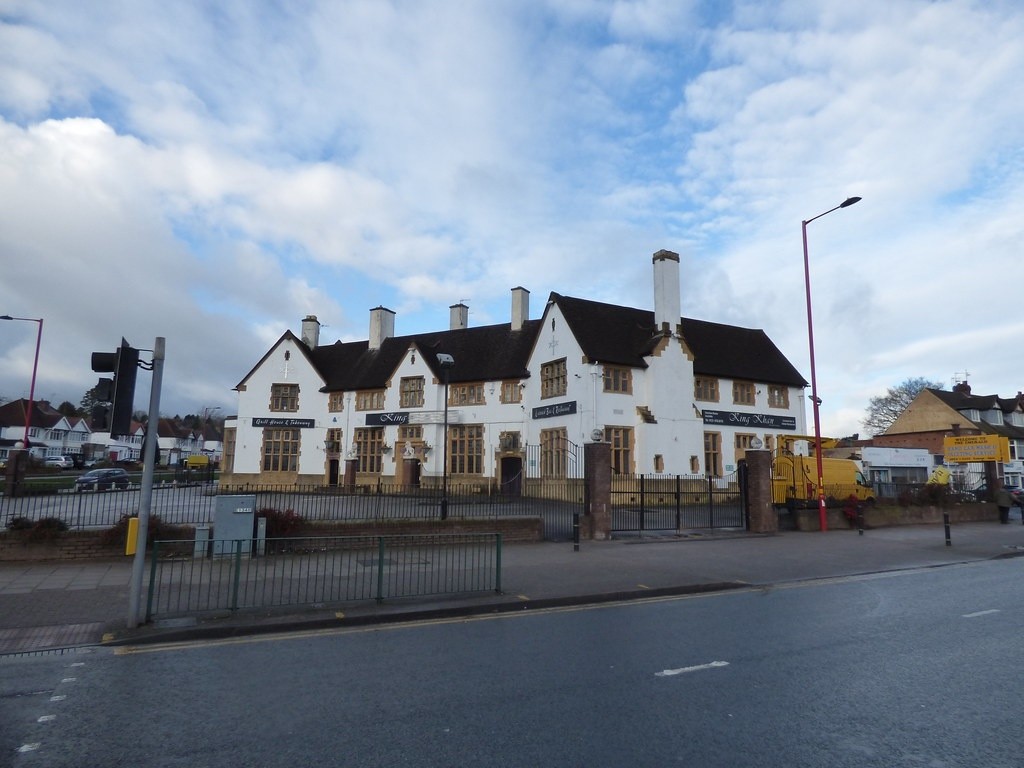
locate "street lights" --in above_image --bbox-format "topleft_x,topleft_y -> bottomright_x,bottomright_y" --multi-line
435,352 -> 456,518
0,315 -> 43,449
801,194 -> 864,530
201,407 -> 222,455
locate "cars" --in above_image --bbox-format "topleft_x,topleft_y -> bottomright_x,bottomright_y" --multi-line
902,483 -> 976,504
83,457 -> 114,468
116,457 -> 143,467
0,457 -> 9,470
75,468 -> 130,492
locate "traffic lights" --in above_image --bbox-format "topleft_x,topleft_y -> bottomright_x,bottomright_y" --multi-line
89,336 -> 139,441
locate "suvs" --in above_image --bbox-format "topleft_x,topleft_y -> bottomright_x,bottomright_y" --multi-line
969,483 -> 1024,504
40,455 -> 74,469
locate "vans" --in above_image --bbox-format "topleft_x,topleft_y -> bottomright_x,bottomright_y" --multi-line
774,456 -> 875,503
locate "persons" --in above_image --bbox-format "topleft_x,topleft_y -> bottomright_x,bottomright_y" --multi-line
994,484 -> 1022,524
